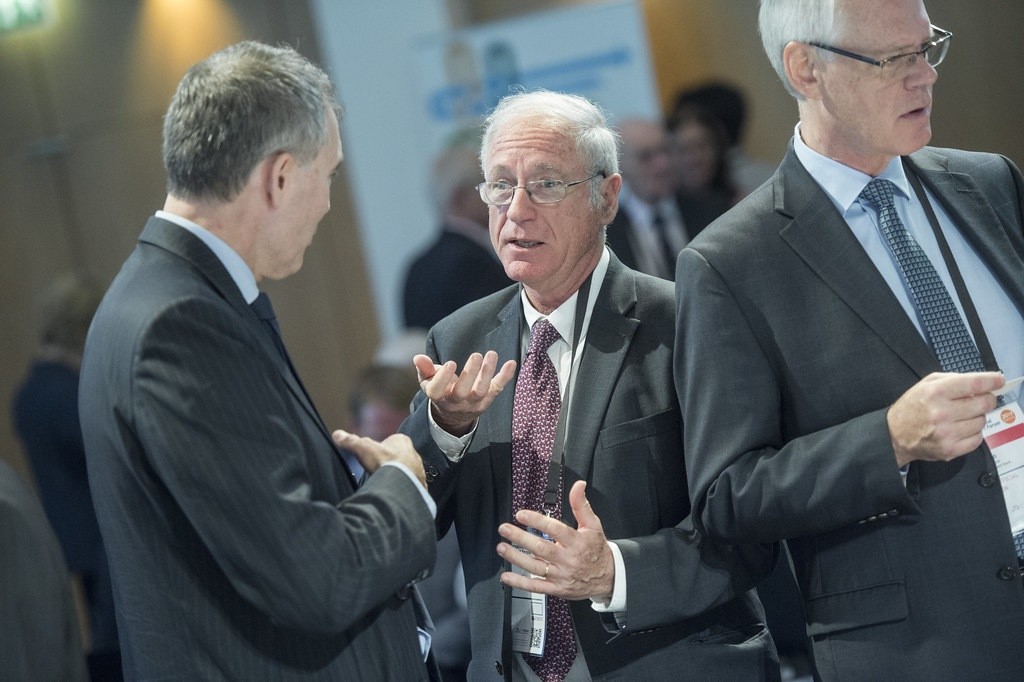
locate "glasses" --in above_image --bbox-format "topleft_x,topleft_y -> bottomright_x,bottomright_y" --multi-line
476,170 -> 607,206
805,24 -> 953,80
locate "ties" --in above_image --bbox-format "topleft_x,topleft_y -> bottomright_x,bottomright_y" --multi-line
249,290 -> 281,341
511,320 -> 578,682
652,216 -> 677,281
858,178 -> 1023,563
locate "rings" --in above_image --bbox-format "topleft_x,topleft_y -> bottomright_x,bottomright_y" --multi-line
543,563 -> 549,578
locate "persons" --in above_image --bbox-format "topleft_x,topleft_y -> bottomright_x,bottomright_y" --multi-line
398,92 -> 783,682
602,77 -> 780,282
9,273 -> 123,681
400,120 -> 520,334
76,38 -> 438,682
672,0 -> 1024,682
336,361 -> 472,681
